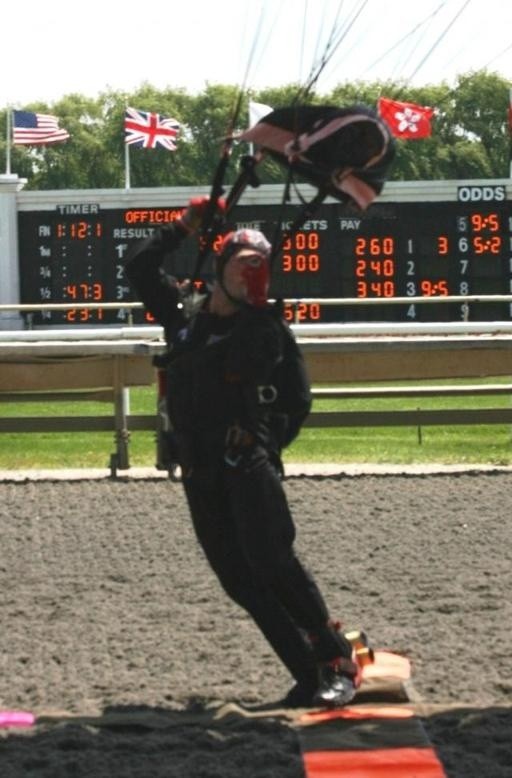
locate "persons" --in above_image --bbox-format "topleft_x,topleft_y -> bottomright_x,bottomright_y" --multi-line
126,197 -> 361,707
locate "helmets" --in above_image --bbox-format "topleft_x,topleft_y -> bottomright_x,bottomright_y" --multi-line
212,228 -> 272,275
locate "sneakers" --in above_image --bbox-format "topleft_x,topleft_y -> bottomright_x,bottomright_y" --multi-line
286,668 -> 356,706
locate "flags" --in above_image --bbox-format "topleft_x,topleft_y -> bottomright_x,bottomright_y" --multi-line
248,102 -> 274,130
377,97 -> 434,140
124,106 -> 179,151
11,110 -> 70,144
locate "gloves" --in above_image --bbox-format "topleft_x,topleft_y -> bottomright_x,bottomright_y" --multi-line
177,196 -> 227,233
